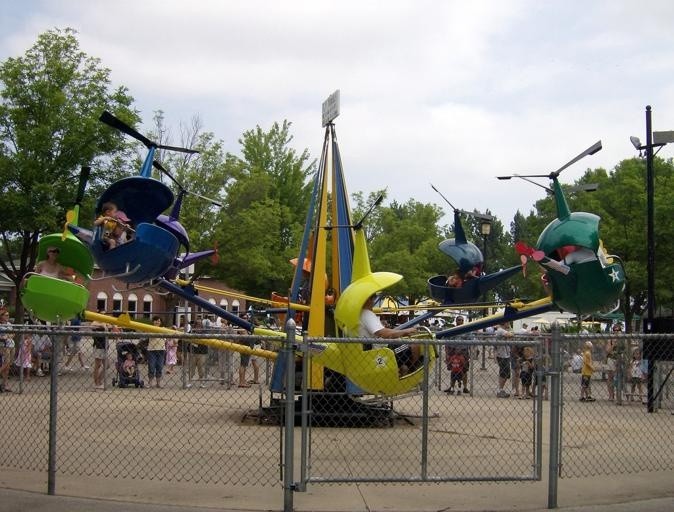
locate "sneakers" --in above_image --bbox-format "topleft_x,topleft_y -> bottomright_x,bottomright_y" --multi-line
33,363 -> 103,389
147,375 -> 263,389
441,386 -> 597,404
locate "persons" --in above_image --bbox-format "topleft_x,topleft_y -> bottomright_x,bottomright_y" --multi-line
92,202 -> 122,251
1,314 -> 279,392
447,271 -> 462,288
34,246 -> 64,279
359,293 -> 431,374
472,264 -> 480,277
115,210 -> 132,246
444,314 -> 643,401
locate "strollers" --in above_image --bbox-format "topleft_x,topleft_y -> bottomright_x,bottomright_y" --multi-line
112,342 -> 144,388
